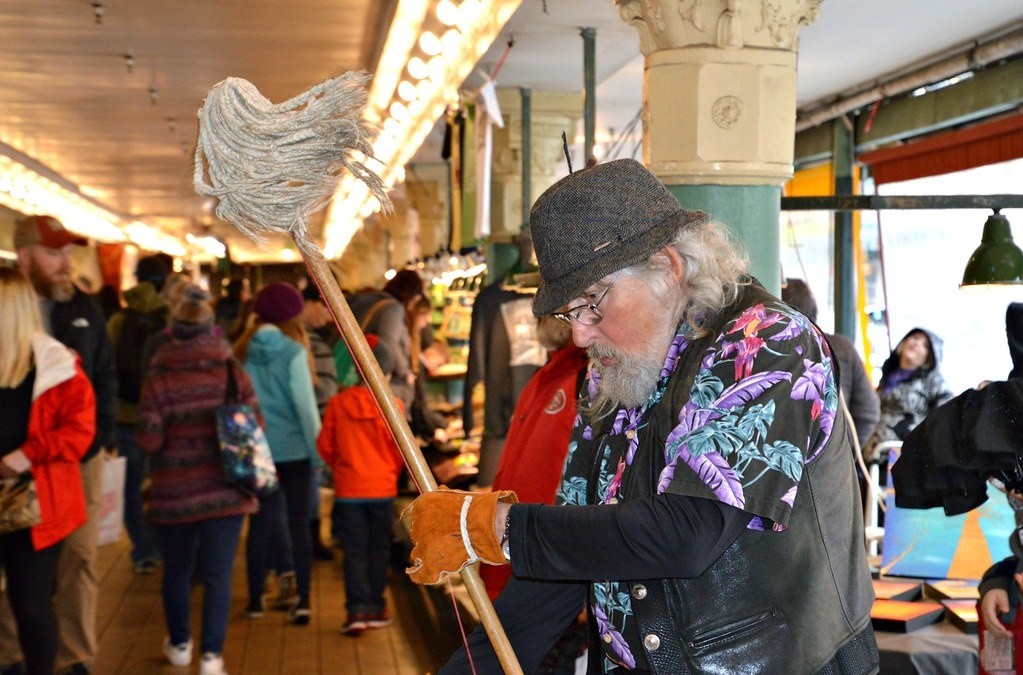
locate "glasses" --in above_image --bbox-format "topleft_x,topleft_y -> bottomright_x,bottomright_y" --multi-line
552,276 -> 616,330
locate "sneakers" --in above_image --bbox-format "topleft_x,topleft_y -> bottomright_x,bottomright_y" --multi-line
161,634 -> 193,667
366,608 -> 394,629
200,653 -> 226,675
339,614 -> 368,635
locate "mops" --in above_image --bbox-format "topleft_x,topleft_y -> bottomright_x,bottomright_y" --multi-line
191,68 -> 526,675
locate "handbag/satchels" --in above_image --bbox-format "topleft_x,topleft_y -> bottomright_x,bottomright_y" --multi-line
331,297 -> 399,387
0,469 -> 39,532
97,454 -> 126,545
211,358 -> 281,499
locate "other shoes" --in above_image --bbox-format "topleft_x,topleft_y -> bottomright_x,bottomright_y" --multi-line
267,571 -> 300,610
242,603 -> 266,619
288,603 -> 313,626
131,556 -> 156,575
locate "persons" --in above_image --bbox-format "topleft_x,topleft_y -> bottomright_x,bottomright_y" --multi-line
134,282 -> 266,675
401,159 -> 880,674
858,327 -> 953,479
0,266 -> 97,675
978,494 -> 1023,639
782,278 -> 880,453
478,306 -> 586,675
0,214 -> 110,675
317,341 -> 405,635
108,249 -> 436,610
231,282 -> 323,627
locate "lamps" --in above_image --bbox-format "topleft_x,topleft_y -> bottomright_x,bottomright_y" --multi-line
957,209 -> 1023,289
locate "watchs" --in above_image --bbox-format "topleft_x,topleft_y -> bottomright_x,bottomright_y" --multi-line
501,509 -> 510,562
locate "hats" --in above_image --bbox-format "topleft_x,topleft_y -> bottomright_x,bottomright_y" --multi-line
782,279 -> 816,318
170,288 -> 216,341
13,215 -> 88,250
252,282 -> 305,326
529,158 -> 711,323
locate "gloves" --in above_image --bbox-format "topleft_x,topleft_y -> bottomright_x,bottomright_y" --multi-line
399,484 -> 520,585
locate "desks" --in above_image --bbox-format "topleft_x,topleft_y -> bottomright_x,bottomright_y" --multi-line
868,591 -> 980,674
